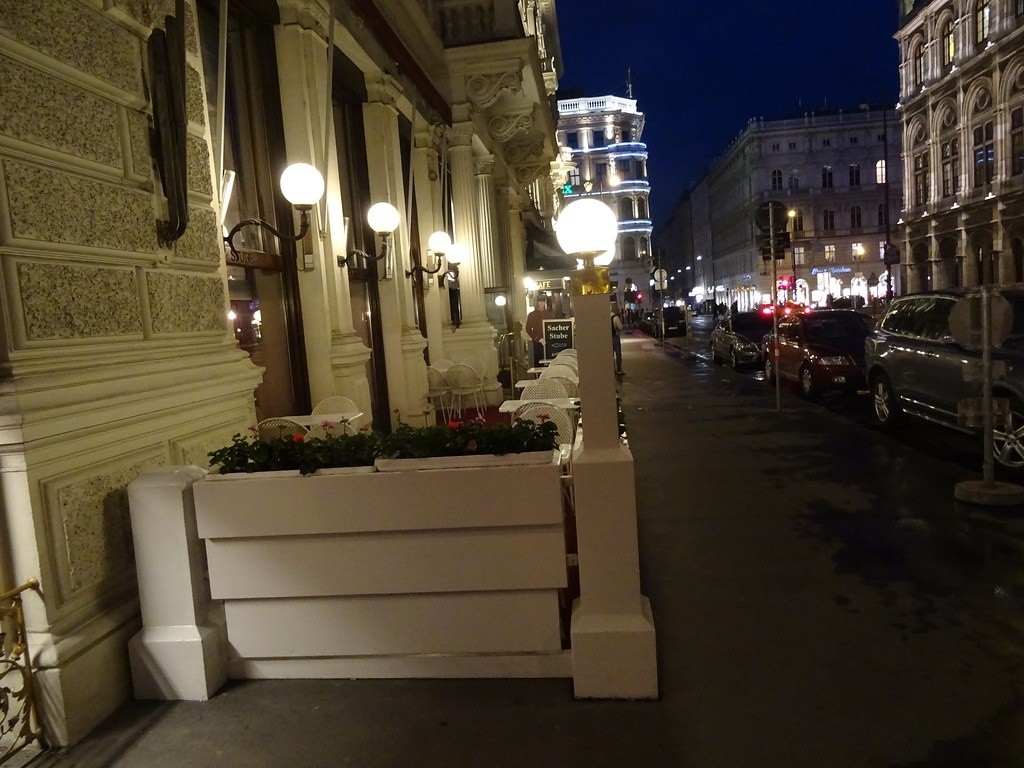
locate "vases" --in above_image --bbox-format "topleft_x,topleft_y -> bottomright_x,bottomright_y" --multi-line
206,465 -> 375,482
374,448 -> 554,472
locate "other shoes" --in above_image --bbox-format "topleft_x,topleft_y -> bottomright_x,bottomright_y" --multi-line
618,371 -> 626,375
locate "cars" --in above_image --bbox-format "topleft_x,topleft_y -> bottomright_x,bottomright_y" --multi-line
634,303 -> 696,339
761,308 -> 878,401
708,311 -> 774,370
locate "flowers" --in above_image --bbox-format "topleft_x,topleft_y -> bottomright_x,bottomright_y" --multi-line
207,418 -> 376,475
376,407 -> 560,459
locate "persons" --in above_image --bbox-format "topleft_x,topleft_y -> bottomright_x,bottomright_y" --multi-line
826,293 -> 836,310
610,305 -> 627,376
527,296 -> 556,379
711,300 -> 739,320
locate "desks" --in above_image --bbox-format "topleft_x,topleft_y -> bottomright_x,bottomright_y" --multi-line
515,377 -> 579,388
259,412 -> 363,428
526,367 -> 578,379
539,359 -> 554,366
498,398 -> 580,413
554,444 -> 572,486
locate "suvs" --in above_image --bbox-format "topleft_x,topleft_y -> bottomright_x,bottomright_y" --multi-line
864,284 -> 1023,481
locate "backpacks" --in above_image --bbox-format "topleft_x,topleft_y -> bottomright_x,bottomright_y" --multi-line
610,314 -> 617,336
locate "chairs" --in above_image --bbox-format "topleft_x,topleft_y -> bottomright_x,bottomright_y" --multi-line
303,422 -> 357,446
539,349 -> 578,377
549,376 -> 577,398
426,356 -> 487,424
512,406 -> 573,508
520,379 -> 576,432
511,401 -> 570,474
258,418 -> 309,443
309,395 -> 359,429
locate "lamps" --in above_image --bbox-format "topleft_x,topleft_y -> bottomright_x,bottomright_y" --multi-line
405,230 -> 451,279
336,200 -> 400,267
556,199 -> 618,295
524,277 -> 537,298
221,161 -> 325,262
438,245 -> 465,290
